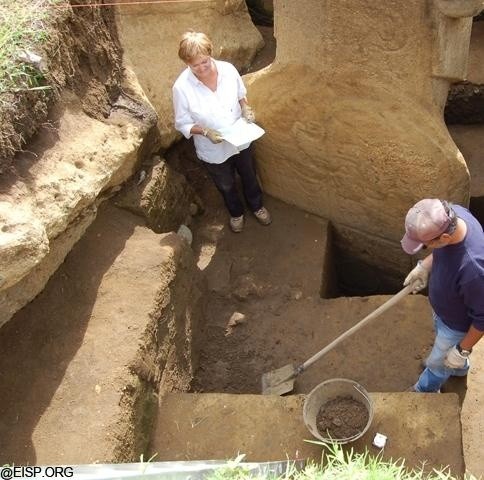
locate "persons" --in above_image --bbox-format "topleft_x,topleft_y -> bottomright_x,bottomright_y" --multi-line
171,30 -> 276,233
399,196 -> 484,394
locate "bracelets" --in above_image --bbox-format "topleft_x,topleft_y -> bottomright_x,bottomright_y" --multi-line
202,127 -> 211,137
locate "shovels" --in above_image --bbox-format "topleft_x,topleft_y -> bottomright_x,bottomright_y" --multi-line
261,279 -> 423,395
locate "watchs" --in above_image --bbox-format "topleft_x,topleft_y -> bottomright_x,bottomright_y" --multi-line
456,343 -> 473,356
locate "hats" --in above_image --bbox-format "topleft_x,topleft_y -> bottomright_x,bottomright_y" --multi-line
400,198 -> 453,256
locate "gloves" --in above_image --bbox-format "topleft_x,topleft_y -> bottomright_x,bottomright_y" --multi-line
201,127 -> 223,144
402,260 -> 429,295
444,344 -> 466,370
241,105 -> 256,122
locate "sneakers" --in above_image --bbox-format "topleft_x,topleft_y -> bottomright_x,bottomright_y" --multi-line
230,214 -> 244,233
253,206 -> 271,225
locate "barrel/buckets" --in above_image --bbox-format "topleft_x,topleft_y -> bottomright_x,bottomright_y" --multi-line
301,376 -> 375,447
